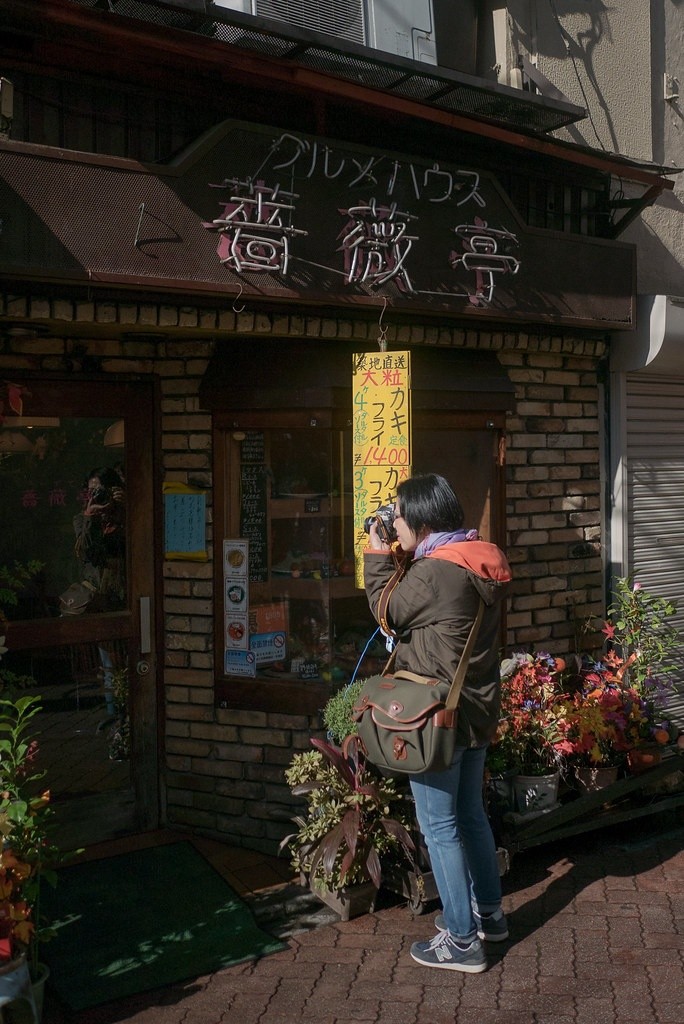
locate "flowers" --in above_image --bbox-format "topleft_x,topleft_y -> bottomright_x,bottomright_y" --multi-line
88,647 -> 130,759
498,647 -> 567,716
508,708 -> 579,772
567,575 -> 684,762
0,695 -> 85,970
483,716 -> 525,783
269,746 -> 416,898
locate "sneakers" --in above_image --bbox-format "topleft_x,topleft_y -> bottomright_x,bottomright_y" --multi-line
435,908 -> 509,941
410,928 -> 488,973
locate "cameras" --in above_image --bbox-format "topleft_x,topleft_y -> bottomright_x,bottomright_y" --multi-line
364,506 -> 399,545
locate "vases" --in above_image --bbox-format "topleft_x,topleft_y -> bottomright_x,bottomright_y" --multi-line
512,764 -> 560,815
22,964 -> 52,1019
557,701 -> 620,766
572,763 -> 617,797
483,772 -> 513,815
625,746 -> 661,770
298,871 -> 379,924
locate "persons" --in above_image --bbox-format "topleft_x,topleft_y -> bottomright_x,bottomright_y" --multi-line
72,467 -> 128,762
363,473 -> 513,974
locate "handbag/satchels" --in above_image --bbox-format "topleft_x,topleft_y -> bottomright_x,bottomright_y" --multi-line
343,676 -> 465,773
56,583 -> 99,617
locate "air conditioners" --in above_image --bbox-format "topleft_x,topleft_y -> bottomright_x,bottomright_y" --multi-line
213,0 -> 440,98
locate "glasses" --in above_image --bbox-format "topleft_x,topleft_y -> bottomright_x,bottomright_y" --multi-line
390,510 -> 401,521
